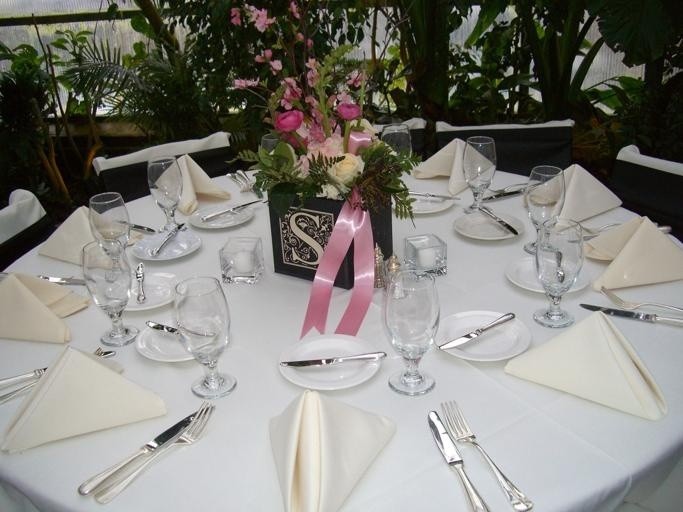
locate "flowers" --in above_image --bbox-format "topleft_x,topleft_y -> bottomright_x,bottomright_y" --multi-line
233,1 -> 420,231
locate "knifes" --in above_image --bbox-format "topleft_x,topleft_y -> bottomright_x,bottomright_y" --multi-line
280,351 -> 385,367
0,348 -> 115,387
200,199 -> 265,223
482,183 -> 540,202
427,408 -> 489,510
117,220 -> 157,234
153,223 -> 183,256
134,264 -> 146,305
77,405 -> 210,496
477,206 -> 520,238
35,275 -> 86,287
405,191 -> 462,202
578,302 -> 682,325
440,313 -> 515,351
568,226 -> 672,244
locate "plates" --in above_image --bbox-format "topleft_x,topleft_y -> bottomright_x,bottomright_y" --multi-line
190,206 -> 252,230
433,309 -> 532,361
129,271 -> 180,310
279,334 -> 381,392
133,232 -> 203,261
134,321 -> 225,361
507,258 -> 593,296
390,193 -> 453,214
456,212 -> 525,241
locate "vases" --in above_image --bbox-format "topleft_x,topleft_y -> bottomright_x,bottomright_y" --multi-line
257,182 -> 397,286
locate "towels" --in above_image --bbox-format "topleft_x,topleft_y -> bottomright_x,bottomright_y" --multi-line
159,154 -> 226,211
507,311 -> 670,419
0,265 -> 88,341
526,164 -> 620,225
268,389 -> 397,511
2,344 -> 174,455
414,139 -> 495,191
579,216 -> 683,292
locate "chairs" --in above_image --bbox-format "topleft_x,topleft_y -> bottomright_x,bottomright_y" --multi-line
0,182 -> 59,259
606,139 -> 683,233
91,132 -> 233,196
432,118 -> 579,189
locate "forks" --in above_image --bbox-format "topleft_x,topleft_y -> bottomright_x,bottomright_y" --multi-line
490,183 -> 529,194
573,220 -> 619,234
95,400 -> 216,505
0,344 -> 103,404
597,286 -> 682,314
226,170 -> 257,193
438,400 -> 534,510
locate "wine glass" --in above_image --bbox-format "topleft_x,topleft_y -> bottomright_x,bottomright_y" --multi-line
149,155 -> 190,234
527,165 -> 567,254
535,219 -> 585,329
174,275 -> 237,401
86,193 -> 134,281
461,136 -> 495,215
383,269 -> 437,396
79,238 -> 139,345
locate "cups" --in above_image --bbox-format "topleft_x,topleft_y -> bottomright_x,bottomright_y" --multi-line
257,135 -> 282,165
379,124 -> 414,165
